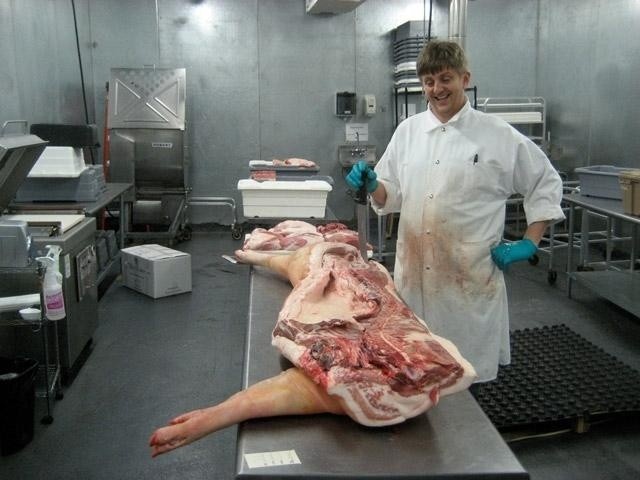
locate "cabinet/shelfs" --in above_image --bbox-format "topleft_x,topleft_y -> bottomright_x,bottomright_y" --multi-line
393,85 -> 477,126
472,97 -> 547,244
529,193 -> 640,319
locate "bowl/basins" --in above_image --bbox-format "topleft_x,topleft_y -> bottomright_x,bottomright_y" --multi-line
18,309 -> 42,323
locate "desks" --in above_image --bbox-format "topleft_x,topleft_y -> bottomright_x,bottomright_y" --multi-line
8,183 -> 135,287
248,204 -> 339,225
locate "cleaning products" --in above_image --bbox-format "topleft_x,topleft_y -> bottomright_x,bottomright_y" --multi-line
35,257 -> 67,321
45,245 -> 64,284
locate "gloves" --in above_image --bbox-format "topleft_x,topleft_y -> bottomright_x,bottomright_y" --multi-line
345,160 -> 378,195
491,238 -> 538,274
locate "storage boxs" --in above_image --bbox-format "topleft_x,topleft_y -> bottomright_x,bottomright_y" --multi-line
619,171 -> 640,216
121,244 -> 192,299
574,166 -> 630,200
238,179 -> 332,218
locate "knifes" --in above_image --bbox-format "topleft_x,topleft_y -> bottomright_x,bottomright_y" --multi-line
353,172 -> 369,264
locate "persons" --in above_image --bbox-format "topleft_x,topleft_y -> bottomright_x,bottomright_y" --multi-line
346,39 -> 566,399
